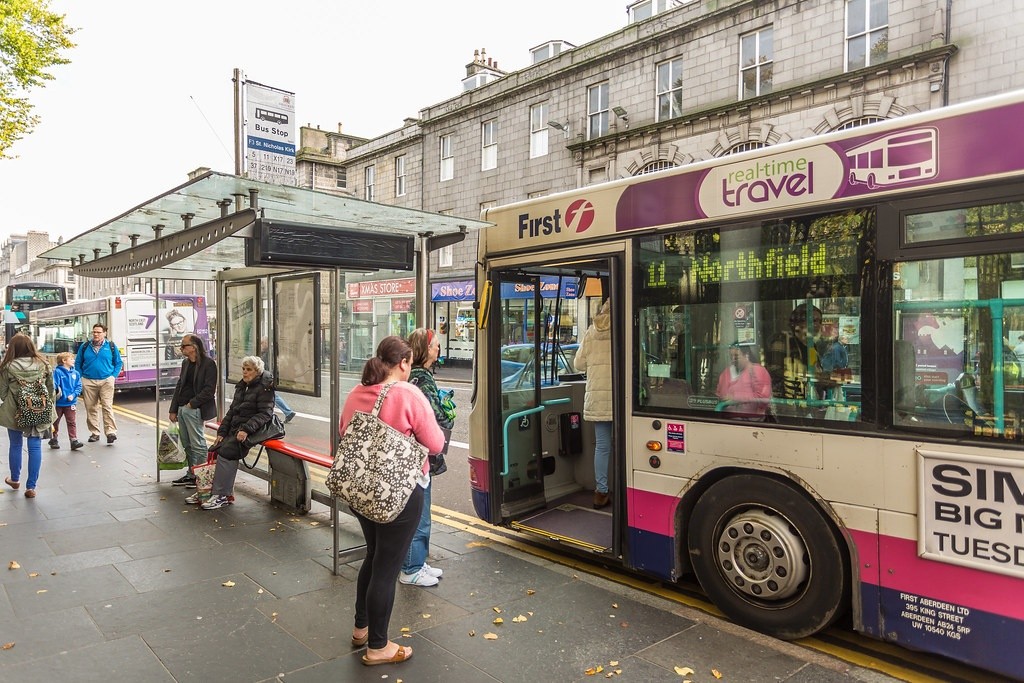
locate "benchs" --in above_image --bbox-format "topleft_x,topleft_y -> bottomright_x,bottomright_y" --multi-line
204,421 -> 334,469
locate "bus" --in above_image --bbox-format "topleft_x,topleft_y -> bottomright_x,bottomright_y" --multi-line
467,89 -> 1024,683
0,280 -> 212,395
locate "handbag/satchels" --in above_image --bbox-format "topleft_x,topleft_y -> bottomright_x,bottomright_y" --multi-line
191,451 -> 235,507
157,419 -> 189,470
422,389 -> 458,420
325,379 -> 430,524
235,412 -> 285,469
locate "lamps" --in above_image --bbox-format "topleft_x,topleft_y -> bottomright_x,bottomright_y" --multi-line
547,117 -> 569,131
612,106 -> 629,122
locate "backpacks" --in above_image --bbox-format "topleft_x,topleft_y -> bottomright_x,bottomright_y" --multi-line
5,362 -> 53,428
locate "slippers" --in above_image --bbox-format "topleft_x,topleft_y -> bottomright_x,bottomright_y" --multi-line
361,645 -> 413,665
352,628 -> 369,647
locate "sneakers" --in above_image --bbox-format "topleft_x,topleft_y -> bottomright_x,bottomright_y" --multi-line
185,492 -> 199,503
421,562 -> 443,577
185,483 -> 196,488
399,569 -> 439,586
172,474 -> 194,485
201,494 -> 228,510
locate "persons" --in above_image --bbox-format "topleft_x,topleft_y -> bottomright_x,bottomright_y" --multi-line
823,335 -> 849,400
716,341 -> 773,422
398,328 -> 455,586
574,297 -> 651,510
670,305 -> 692,380
169,334 -> 218,487
48,352 -> 83,450
185,356 -> 275,510
0,334 -> 58,498
75,323 -> 123,443
339,336 -> 447,665
275,393 -> 296,423
1004,334 -> 1024,385
767,303 -> 823,417
165,310 -> 193,360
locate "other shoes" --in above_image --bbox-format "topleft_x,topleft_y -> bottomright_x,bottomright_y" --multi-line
71,441 -> 84,451
592,490 -> 610,510
88,434 -> 100,442
25,489 -> 36,498
284,411 -> 296,424
106,433 -> 117,443
4,476 -> 20,489
48,438 -> 60,449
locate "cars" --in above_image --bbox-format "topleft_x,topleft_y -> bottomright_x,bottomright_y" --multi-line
501,341 -> 665,391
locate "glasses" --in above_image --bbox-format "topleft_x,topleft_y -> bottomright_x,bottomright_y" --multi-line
180,343 -> 193,349
